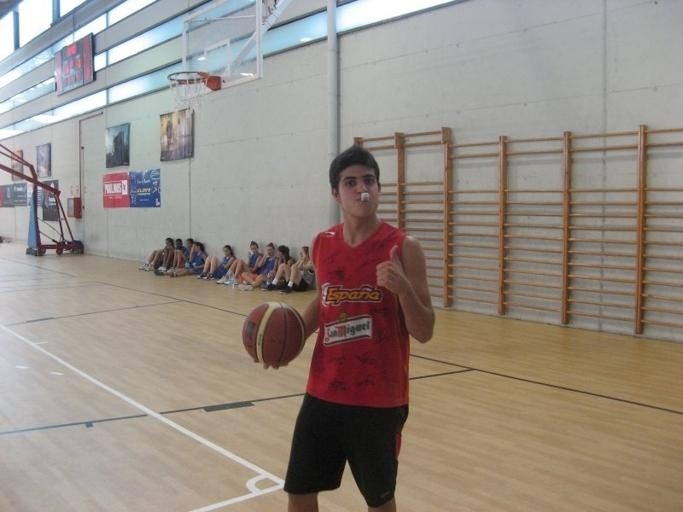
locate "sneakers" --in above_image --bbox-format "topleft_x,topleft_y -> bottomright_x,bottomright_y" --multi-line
138,263 -> 176,277
197,273 -> 214,280
216,276 -> 291,294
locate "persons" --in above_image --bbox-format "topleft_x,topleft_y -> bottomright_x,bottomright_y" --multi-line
251,146 -> 435,511
136,236 -> 315,293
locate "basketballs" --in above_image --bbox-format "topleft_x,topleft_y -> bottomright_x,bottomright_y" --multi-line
243,302 -> 305,366
